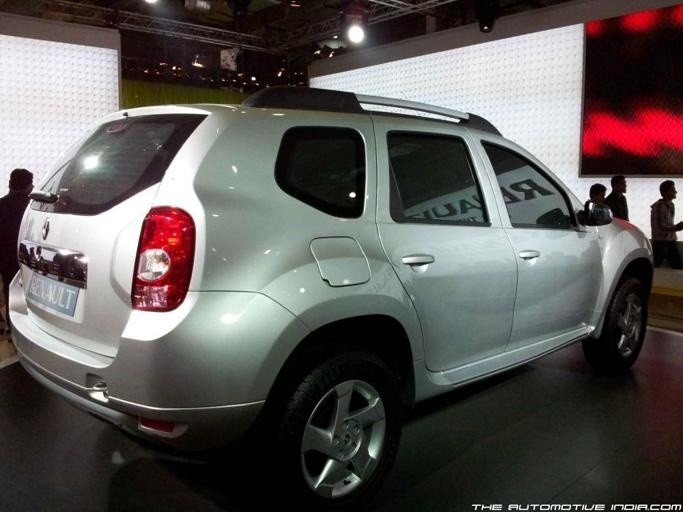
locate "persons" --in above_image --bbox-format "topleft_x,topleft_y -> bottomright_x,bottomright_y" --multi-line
650,180 -> 683,270
590,183 -> 606,202
605,176 -> 628,221
1,169 -> 34,331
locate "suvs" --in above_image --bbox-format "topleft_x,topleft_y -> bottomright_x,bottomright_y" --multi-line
6,86 -> 655,511
